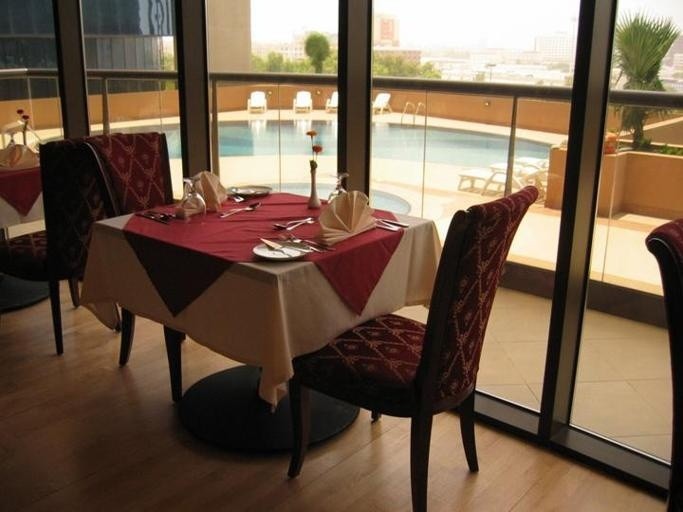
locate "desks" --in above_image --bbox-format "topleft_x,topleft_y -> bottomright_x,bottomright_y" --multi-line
81,182 -> 438,480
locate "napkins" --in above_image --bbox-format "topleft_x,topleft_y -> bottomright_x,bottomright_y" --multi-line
318,191 -> 378,243
186,173 -> 230,212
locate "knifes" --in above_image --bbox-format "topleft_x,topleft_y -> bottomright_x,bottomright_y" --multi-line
257,235 -> 302,258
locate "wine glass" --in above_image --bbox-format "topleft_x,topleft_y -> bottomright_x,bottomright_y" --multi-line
181,176 -> 207,225
326,172 -> 349,206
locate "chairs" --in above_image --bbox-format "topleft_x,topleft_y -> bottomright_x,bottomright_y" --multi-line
69,131 -> 199,396
0,139 -> 68,356
645,220 -> 683,512
291,187 -> 542,511
245,90 -> 395,116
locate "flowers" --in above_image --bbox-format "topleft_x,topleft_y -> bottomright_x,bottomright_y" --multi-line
16,108 -> 30,132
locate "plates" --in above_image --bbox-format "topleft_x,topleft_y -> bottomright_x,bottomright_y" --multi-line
252,240 -> 311,262
228,185 -> 272,197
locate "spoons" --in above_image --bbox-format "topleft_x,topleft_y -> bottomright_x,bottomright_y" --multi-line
219,201 -> 261,218
272,219 -> 316,229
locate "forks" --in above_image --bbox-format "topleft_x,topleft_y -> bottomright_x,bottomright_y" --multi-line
288,231 -> 337,252
